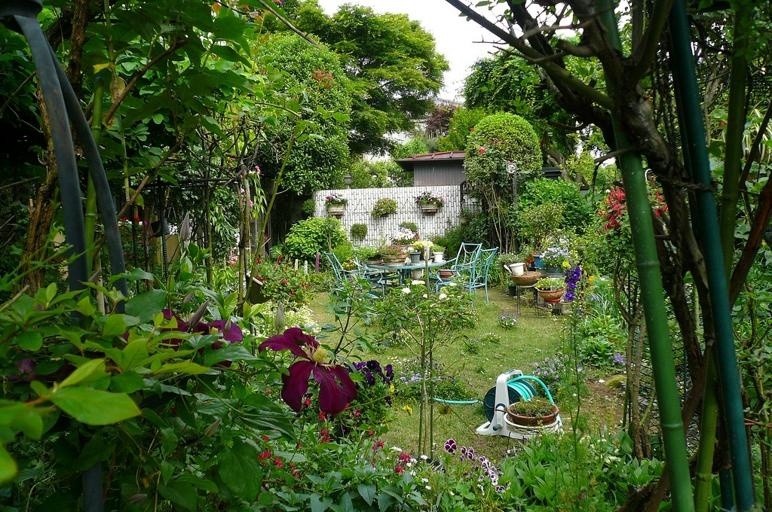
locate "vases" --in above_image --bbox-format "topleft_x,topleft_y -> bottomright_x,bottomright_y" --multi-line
328,204 -> 345,215
421,203 -> 438,213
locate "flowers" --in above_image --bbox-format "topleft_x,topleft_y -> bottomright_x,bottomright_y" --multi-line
413,190 -> 445,208
325,193 -> 348,210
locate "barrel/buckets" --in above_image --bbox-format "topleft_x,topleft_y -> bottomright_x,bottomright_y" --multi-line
410,268 -> 423,279
509,263 -> 525,275
432,251 -> 444,262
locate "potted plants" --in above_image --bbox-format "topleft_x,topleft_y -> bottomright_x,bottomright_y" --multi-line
532,250 -> 571,303
366,222 -> 444,266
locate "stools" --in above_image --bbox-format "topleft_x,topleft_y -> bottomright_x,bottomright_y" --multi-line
517,284 -> 539,317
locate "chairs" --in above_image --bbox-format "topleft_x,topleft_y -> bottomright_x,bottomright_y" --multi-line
324,248 -> 397,314
434,241 -> 500,304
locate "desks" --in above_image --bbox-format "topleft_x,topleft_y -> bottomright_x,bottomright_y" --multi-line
506,396 -> 560,427
369,259 -> 447,285
